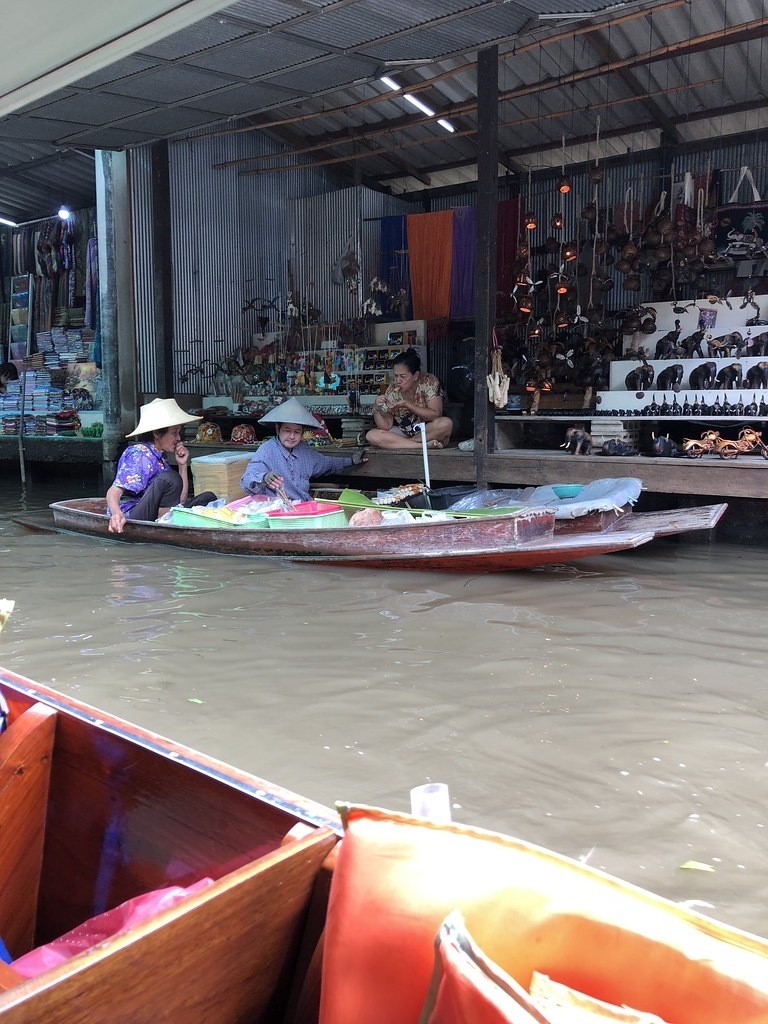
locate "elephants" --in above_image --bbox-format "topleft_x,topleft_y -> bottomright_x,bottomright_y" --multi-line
559,428 -> 592,456
642,394 -> 768,416
601,431 -> 678,457
624,319 -> 768,390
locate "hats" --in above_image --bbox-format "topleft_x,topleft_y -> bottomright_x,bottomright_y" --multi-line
258,396 -> 325,430
125,397 -> 204,438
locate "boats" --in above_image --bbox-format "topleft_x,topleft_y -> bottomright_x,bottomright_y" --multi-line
12,498 -> 728,573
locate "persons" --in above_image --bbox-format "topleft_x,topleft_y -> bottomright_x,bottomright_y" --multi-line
0,362 -> 20,393
106,396 -> 220,534
240,397 -> 368,503
364,346 -> 452,450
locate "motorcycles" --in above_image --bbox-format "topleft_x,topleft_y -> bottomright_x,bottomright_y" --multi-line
712,424 -> 768,462
681,430 -> 728,458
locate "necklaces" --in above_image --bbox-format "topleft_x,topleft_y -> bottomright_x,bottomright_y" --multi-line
268,321 -> 340,388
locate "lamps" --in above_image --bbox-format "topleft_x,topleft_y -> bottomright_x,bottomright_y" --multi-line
508,0 -> 768,393
56,151 -> 70,220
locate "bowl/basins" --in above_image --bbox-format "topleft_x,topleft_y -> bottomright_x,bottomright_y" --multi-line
552,484 -> 583,498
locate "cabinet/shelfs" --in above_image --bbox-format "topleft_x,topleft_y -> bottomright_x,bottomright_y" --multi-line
202,320 -> 427,417
596,294 -> 768,421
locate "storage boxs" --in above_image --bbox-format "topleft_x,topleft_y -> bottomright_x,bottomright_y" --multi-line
190,449 -> 257,504
265,499 -> 350,531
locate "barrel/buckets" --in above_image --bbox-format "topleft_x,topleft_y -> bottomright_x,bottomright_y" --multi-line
417,476 -> 479,511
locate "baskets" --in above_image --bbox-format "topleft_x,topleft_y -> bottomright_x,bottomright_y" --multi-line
169,494 -> 350,528
